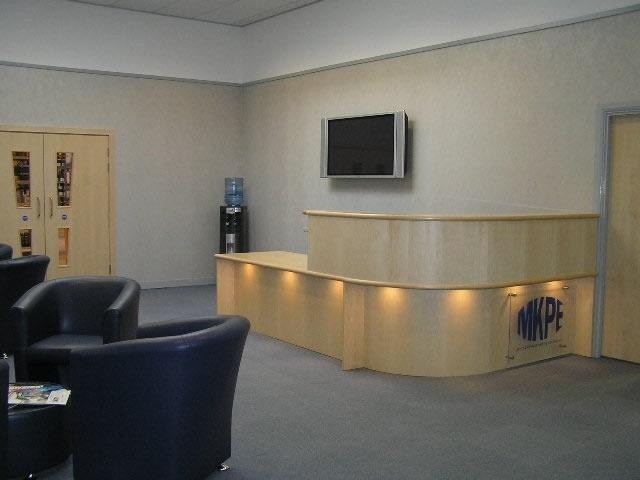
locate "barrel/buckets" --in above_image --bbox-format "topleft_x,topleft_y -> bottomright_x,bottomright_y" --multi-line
225,177 -> 243,205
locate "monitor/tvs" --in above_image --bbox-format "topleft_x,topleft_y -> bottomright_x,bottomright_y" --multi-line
320,111 -> 408,179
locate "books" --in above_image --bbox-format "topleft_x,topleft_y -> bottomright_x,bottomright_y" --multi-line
9,383 -> 73,407
